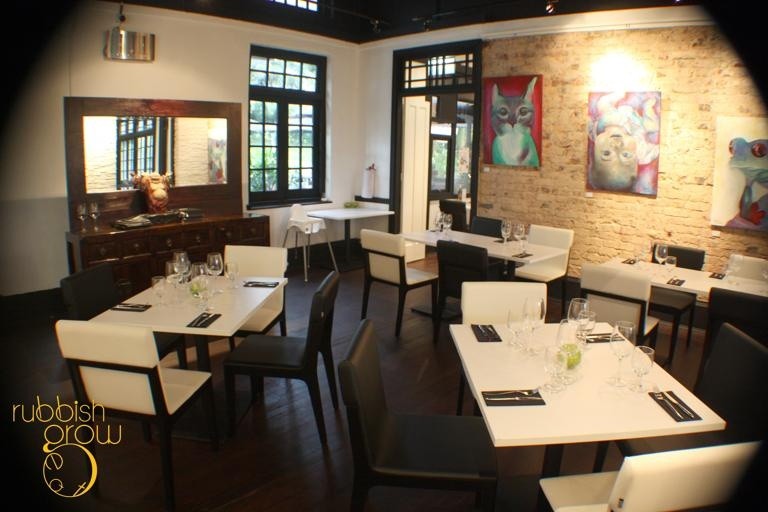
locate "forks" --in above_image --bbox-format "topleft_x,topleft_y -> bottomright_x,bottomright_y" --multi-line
483,388 -> 543,395
653,384 -> 683,421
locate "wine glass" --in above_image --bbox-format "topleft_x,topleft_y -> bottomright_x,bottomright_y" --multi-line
633,240 -> 677,285
76,201 -> 100,234
434,214 -> 452,239
610,320 -> 655,393
508,297 -> 597,394
500,219 -> 525,254
152,250 -> 238,311
729,253 -> 744,286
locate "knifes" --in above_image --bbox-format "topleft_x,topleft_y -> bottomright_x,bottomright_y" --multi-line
663,390 -> 695,419
485,396 -> 542,402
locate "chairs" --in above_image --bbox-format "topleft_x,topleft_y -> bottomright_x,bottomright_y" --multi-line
54,199 -> 768,512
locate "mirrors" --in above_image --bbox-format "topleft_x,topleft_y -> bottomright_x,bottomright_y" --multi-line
64,95 -> 243,230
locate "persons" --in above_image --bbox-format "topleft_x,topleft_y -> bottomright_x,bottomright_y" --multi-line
209,141 -> 224,183
